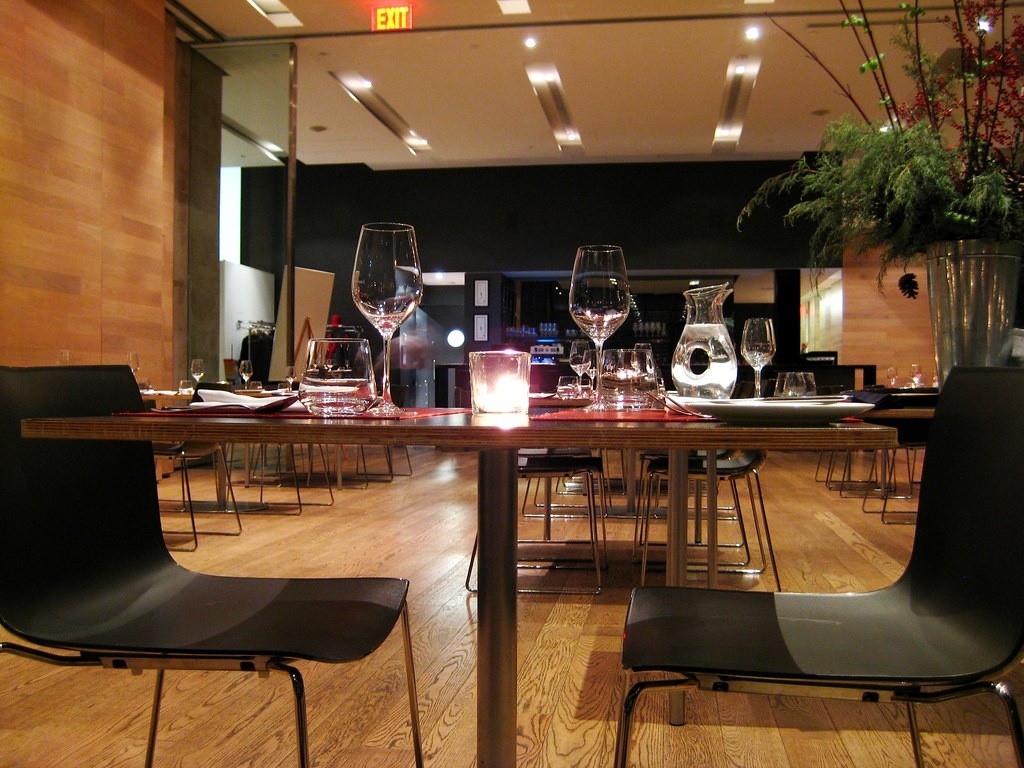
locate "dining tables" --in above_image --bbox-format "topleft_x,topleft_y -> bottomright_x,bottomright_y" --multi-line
21,407 -> 898,768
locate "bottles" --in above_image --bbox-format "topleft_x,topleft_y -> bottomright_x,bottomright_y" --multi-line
566,329 -> 585,337
632,322 -> 666,337
539,322 -> 558,338
648,339 -> 670,365
671,282 -> 738,399
505,325 -> 537,335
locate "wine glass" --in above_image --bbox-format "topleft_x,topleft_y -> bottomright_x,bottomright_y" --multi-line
191,359 -> 204,382
569,341 -> 591,399
887,364 -> 938,389
569,245 -> 630,411
285,366 -> 296,393
136,377 -> 150,398
352,223 -> 423,416
741,319 -> 776,399
239,360 -> 253,390
582,350 -> 598,394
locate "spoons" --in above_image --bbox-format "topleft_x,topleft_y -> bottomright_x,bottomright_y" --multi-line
150,396 -> 298,415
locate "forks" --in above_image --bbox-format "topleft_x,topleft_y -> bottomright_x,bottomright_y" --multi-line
646,391 -> 713,417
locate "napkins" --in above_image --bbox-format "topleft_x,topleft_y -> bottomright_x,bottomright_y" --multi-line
665,395 -> 711,411
189,387 -> 306,411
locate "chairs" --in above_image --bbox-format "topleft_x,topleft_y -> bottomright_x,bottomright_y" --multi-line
614,366 -> 1024,768
0,366 -> 423,768
466,370 -> 625,594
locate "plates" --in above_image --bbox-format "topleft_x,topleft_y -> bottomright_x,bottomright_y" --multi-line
234,390 -> 262,393
684,402 -> 875,425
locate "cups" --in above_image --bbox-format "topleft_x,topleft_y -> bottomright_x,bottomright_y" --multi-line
55,349 -> 75,365
557,376 -> 580,400
179,380 -> 193,394
278,383 -> 288,393
298,338 -> 377,417
127,353 -> 139,373
468,351 -> 532,416
774,372 -> 817,397
601,349 -> 657,412
249,380 -> 262,390
